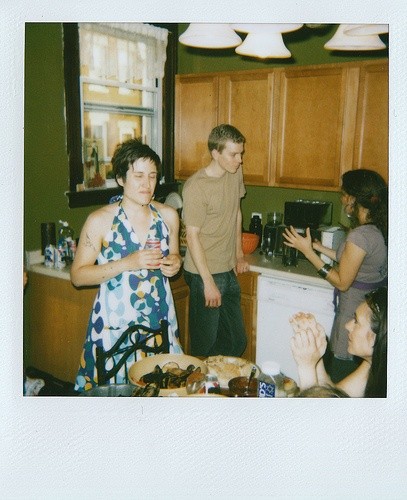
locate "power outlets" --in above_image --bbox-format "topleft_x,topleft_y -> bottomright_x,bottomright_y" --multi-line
251,212 -> 262,219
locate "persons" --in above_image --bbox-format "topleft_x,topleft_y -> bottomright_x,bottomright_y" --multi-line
70,138 -> 184,391
282,169 -> 388,398
181,124 -> 249,359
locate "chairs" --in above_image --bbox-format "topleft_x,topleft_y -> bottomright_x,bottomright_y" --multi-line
96,319 -> 170,388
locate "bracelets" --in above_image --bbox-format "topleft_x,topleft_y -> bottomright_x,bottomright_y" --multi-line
317,263 -> 332,278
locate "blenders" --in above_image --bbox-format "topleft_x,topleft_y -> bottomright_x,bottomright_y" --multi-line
263,211 -> 286,256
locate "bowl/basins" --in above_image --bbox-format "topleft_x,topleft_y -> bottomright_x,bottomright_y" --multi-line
262,374 -> 297,397
128,353 -> 208,395
228,375 -> 258,397
241,233 -> 260,256
203,355 -> 261,390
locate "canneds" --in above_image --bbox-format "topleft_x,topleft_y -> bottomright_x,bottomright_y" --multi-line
44,244 -> 66,268
146,238 -> 161,267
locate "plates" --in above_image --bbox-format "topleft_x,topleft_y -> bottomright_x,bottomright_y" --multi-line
78,383 -> 153,397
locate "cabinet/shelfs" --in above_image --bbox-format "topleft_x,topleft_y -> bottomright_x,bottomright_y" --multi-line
275,57 -> 389,194
174,68 -> 276,188
29,272 -> 190,387
236,270 -> 262,365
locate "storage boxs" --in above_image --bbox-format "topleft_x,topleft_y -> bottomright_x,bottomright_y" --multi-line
321,226 -> 346,267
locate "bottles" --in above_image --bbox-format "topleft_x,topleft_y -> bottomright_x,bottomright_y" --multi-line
58,219 -> 74,248
283,231 -> 297,267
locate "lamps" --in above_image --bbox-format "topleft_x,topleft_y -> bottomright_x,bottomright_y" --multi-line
236,23 -> 304,34
235,23 -> 292,60
341,24 -> 389,35
322,25 -> 386,51
178,23 -> 242,51
